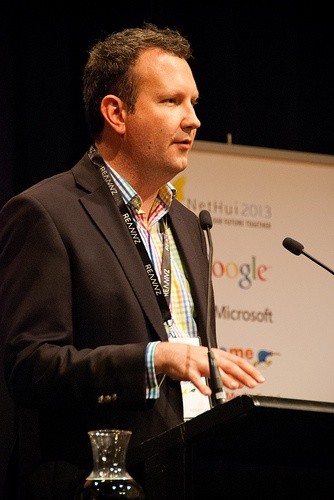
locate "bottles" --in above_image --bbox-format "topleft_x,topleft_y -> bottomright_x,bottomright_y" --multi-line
81,430 -> 144,500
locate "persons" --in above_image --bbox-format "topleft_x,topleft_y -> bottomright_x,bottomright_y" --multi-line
0,24 -> 263,500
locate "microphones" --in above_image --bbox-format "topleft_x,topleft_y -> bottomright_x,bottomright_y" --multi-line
282,237 -> 334,275
199,210 -> 227,408
